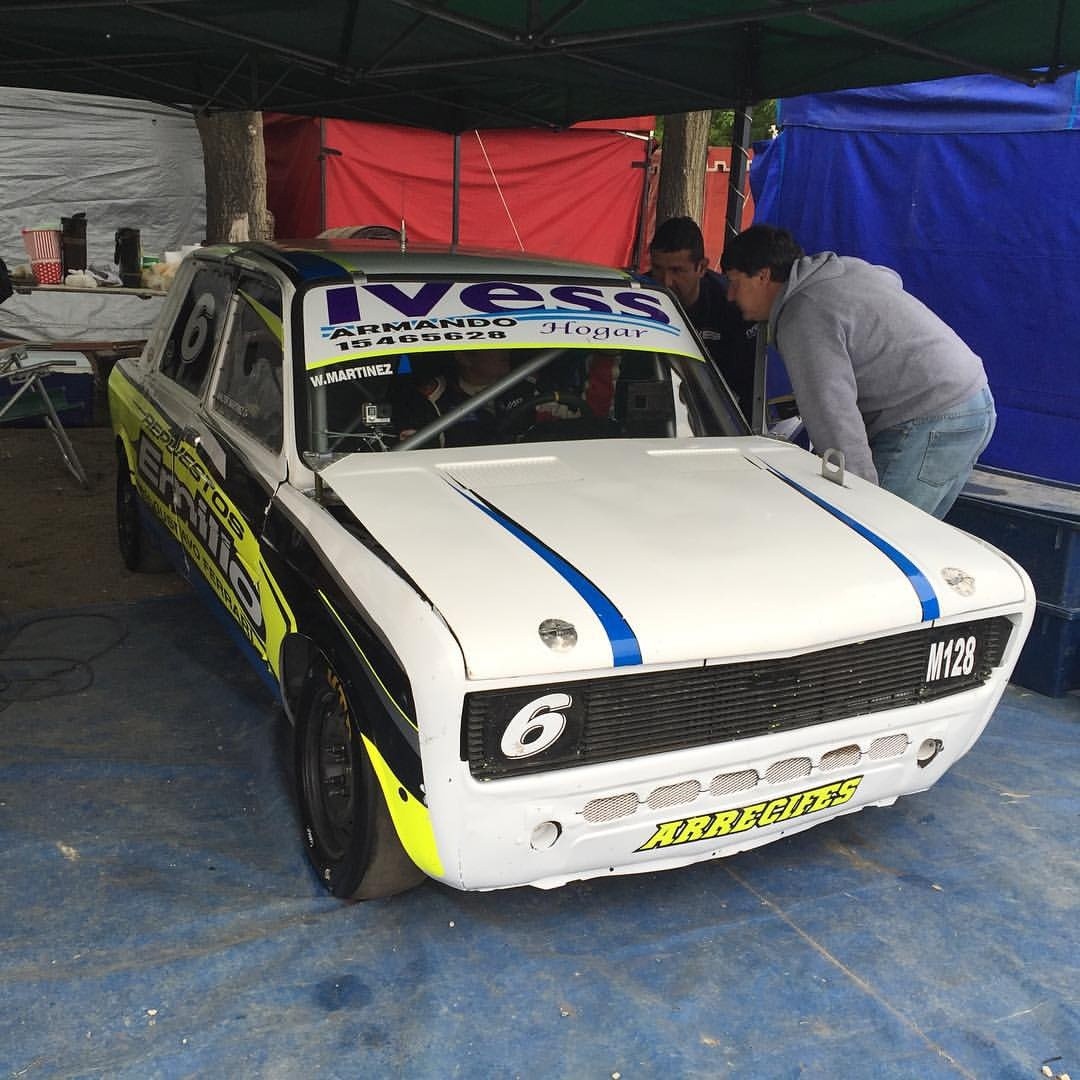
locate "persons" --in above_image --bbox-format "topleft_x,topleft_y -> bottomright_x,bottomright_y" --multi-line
388,350 -> 598,450
615,218 -> 758,436
720,226 -> 997,522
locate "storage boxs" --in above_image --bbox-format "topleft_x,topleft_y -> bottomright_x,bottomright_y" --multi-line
943,487 -> 1080,696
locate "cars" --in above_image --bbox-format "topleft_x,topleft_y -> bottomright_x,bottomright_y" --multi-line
104,243 -> 1036,898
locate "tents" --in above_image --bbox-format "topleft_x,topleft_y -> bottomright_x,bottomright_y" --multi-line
750,64 -> 1080,487
0,89 -> 754,276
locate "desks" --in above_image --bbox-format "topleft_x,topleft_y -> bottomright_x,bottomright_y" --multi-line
0,345 -> 95,487
